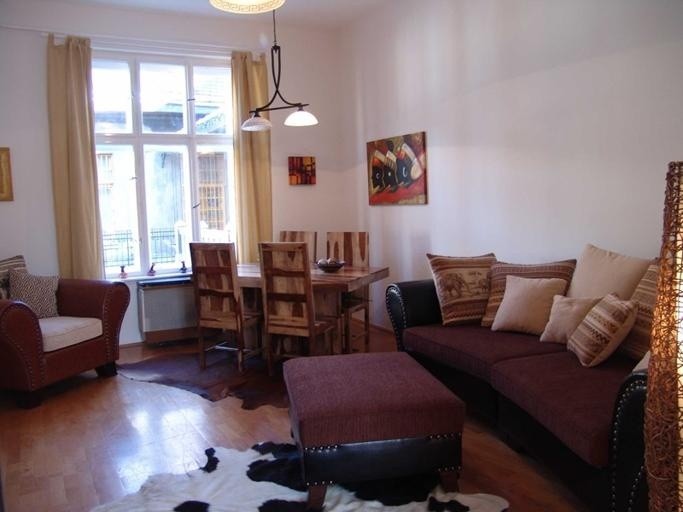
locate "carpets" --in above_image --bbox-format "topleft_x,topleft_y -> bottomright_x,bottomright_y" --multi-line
88,439 -> 509,510
114,348 -> 290,409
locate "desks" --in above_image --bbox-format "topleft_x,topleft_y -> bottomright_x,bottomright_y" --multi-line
224,264 -> 390,354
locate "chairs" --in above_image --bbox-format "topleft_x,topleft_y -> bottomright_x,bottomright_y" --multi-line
187,240 -> 264,376
324,230 -> 370,355
257,241 -> 336,359
278,229 -> 317,264
0,253 -> 130,411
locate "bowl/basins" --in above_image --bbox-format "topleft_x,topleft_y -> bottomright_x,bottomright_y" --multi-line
314,261 -> 345,272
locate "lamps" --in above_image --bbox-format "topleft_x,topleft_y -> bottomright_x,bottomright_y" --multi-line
208,0 -> 286,16
640,159 -> 683,511
239,10 -> 320,134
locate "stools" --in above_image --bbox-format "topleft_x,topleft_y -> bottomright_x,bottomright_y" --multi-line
281,348 -> 467,507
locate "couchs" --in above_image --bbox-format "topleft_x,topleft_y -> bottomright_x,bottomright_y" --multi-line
384,275 -> 651,509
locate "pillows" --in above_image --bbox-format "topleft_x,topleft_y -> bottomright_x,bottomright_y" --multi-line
480,259 -> 577,329
5,266 -> 61,319
490,273 -> 569,334
537,293 -> 602,345
0,255 -> 29,302
566,291 -> 640,368
615,256 -> 661,361
426,252 -> 498,327
566,242 -> 651,300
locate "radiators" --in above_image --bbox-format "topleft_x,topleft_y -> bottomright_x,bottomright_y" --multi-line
135,276 -> 198,341
